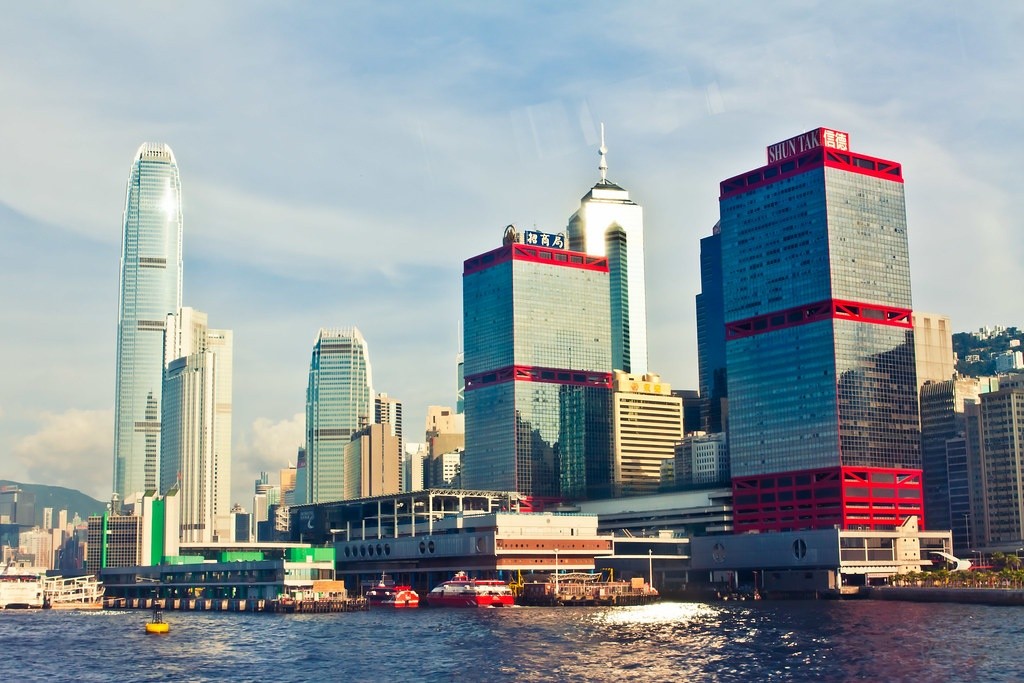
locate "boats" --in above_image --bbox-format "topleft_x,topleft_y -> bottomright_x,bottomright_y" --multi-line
425,569 -> 516,608
146,622 -> 169,634
364,570 -> 420,610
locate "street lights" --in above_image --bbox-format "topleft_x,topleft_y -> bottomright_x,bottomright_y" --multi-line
1015,547 -> 1024,571
972,549 -> 982,572
